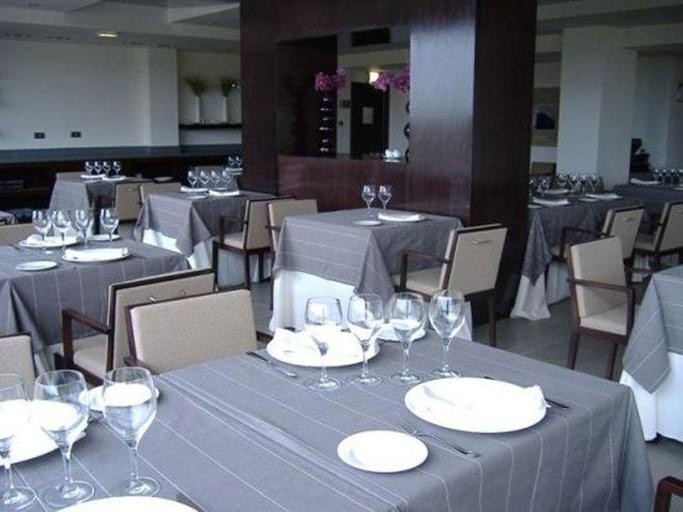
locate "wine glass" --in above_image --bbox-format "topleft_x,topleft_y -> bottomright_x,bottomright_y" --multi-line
186,169 -> 233,200
84,161 -> 121,181
0,373 -> 36,511
226,155 -> 242,167
389,292 -> 426,382
578,172 -> 600,192
427,290 -> 466,377
651,167 -> 683,188
32,370 -> 94,507
377,184 -> 392,211
70,208 -> 93,247
100,208 -> 119,247
31,209 -> 51,255
304,296 -> 342,389
50,208 -> 71,249
528,174 -> 578,197
360,184 -> 375,219
346,294 -> 383,386
102,367 -> 158,496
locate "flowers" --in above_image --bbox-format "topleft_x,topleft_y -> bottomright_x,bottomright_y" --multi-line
313,66 -> 346,92
371,64 -> 410,94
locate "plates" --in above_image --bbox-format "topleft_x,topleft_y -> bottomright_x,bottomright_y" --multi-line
402,376 -> 547,433
88,234 -> 122,241
377,211 -> 424,223
532,197 -> 572,207
266,330 -> 380,368
377,324 -> 425,341
14,259 -> 56,271
16,235 -> 78,249
0,398 -> 85,465
55,496 -> 197,512
585,193 -> 622,202
337,429 -> 429,475
79,384 -> 160,411
351,219 -> 381,226
61,248 -> 132,263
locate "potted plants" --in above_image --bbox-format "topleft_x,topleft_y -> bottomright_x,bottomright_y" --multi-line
218,78 -> 236,124
183,76 -> 205,123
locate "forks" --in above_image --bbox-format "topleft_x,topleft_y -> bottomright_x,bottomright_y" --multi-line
65,398 -> 103,423
393,415 -> 475,458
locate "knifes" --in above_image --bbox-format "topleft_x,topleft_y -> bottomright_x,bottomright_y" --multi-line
246,351 -> 297,379
483,374 -> 571,412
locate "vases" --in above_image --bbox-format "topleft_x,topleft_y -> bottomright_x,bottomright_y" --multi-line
402,86 -> 411,162
315,90 -> 333,159
